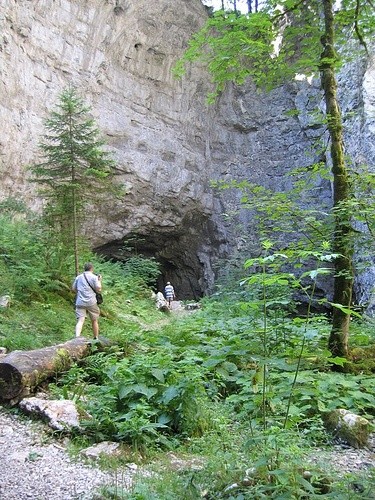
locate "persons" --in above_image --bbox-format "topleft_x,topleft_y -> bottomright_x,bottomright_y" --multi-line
71,263 -> 102,340
164,281 -> 176,306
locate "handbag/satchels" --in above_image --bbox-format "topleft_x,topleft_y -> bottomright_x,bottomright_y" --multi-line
96,293 -> 103,304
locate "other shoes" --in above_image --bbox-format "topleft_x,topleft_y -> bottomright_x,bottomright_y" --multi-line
94,338 -> 99,341
